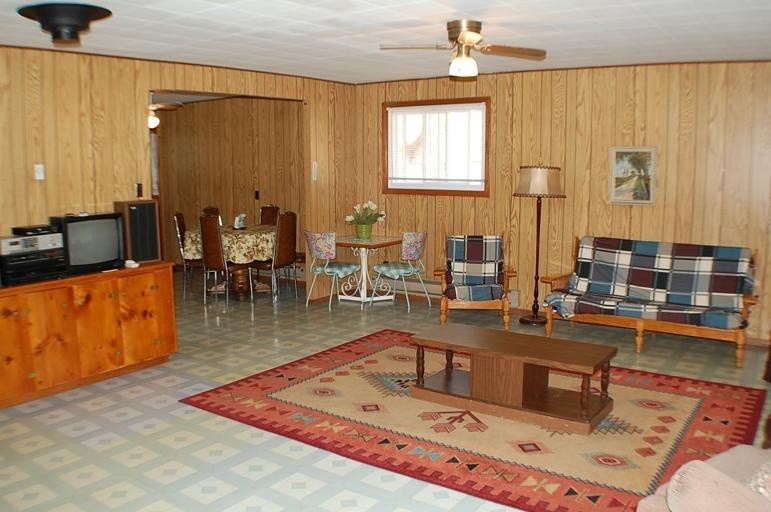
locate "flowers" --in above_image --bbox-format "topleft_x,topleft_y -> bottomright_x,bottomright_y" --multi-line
345,200 -> 387,226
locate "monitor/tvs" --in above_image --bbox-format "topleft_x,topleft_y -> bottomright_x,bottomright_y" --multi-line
50,211 -> 127,273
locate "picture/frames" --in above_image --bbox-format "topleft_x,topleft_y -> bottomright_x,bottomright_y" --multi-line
607,146 -> 657,204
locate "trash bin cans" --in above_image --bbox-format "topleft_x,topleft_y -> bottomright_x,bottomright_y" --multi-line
233,269 -> 250,300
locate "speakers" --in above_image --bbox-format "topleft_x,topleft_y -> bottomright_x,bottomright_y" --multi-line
114,198 -> 162,265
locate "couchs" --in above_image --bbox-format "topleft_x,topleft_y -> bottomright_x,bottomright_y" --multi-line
540,235 -> 759,367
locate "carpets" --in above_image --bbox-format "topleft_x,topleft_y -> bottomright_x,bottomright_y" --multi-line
178,327 -> 766,512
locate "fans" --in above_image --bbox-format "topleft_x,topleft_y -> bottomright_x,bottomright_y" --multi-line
378,18 -> 548,66
147,92 -> 185,136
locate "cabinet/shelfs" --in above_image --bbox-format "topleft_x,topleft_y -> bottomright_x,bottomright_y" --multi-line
0,262 -> 180,409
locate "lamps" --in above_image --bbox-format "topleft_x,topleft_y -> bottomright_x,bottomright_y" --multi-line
147,92 -> 161,130
510,165 -> 567,325
447,54 -> 479,78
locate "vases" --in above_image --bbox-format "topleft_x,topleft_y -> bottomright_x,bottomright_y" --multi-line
355,224 -> 373,239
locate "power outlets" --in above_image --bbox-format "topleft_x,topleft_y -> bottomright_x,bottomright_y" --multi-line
33,162 -> 45,180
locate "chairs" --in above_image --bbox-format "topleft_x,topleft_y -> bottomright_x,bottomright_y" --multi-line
302,230 -> 361,313
369,232 -> 432,313
174,212 -> 218,301
199,214 -> 251,304
433,234 -> 517,329
257,205 -> 281,294
204,207 -> 219,215
250,211 -> 298,302
